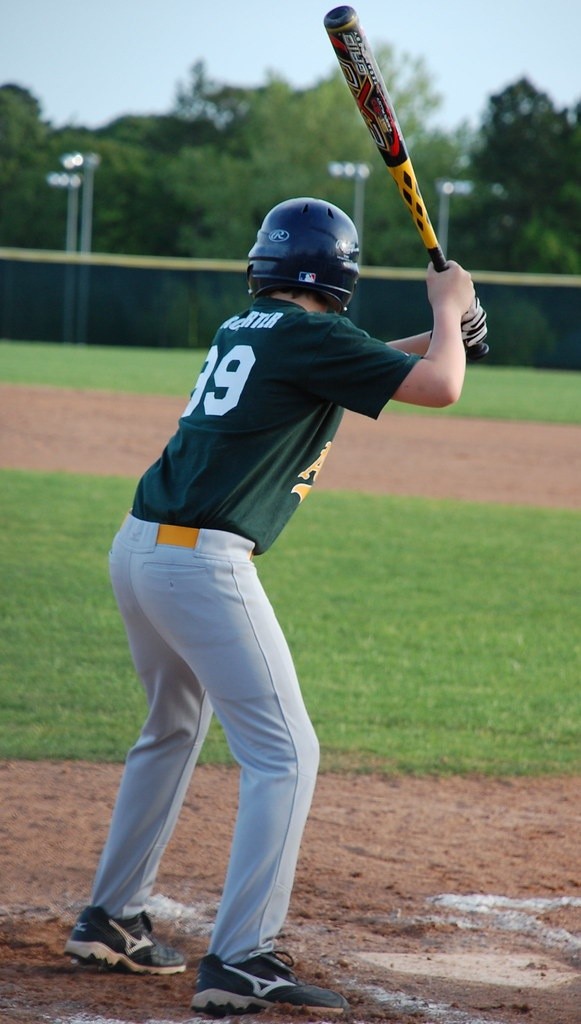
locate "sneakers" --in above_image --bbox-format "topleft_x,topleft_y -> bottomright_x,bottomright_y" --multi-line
64,906 -> 187,975
193,951 -> 350,1018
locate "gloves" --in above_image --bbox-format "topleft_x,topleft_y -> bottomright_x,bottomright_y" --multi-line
429,297 -> 488,352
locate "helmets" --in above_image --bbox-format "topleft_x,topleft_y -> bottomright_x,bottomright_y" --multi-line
247,199 -> 359,316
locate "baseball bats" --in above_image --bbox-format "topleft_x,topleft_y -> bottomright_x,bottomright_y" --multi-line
323,4 -> 491,362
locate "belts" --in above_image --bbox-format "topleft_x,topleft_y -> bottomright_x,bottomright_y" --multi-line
121,508 -> 257,562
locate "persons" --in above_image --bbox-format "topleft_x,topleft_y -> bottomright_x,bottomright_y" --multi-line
64,197 -> 488,1015
